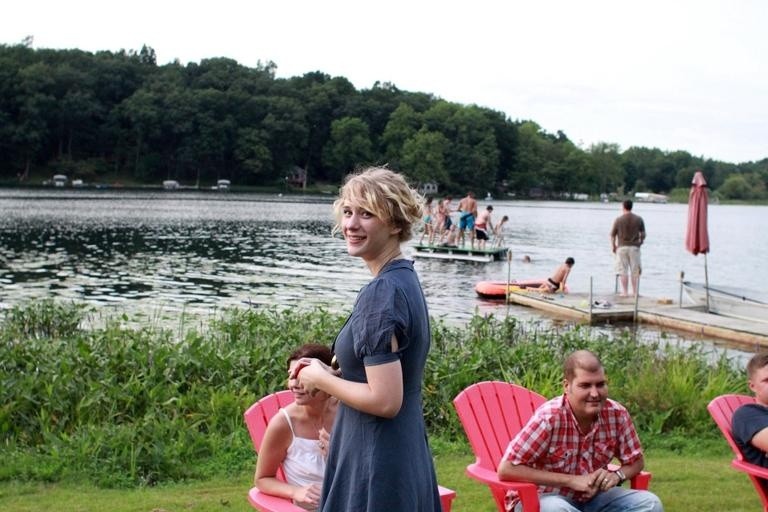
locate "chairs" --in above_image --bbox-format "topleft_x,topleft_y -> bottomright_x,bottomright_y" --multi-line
450,381 -> 651,512
706,393 -> 767,512
243,390 -> 456,512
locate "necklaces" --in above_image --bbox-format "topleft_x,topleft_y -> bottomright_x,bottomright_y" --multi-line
301,404 -> 324,439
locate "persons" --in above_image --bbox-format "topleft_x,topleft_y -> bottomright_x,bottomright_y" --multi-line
294,164 -> 444,511
252,343 -> 344,511
730,353 -> 768,493
420,191 -> 510,248
611,198 -> 648,296
545,257 -> 575,293
501,350 -> 665,511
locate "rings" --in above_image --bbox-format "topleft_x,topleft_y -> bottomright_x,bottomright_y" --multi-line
603,478 -> 609,482
319,442 -> 325,449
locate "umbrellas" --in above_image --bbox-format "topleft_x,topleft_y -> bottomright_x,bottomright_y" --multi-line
686,169 -> 714,312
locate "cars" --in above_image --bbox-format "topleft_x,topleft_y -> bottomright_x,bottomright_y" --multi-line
215,178 -> 231,192
42,175 -> 82,187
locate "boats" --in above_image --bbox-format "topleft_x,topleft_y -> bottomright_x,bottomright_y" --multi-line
473,277 -> 569,303
408,238 -> 512,263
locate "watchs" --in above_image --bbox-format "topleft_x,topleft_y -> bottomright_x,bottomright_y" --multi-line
614,468 -> 627,487
